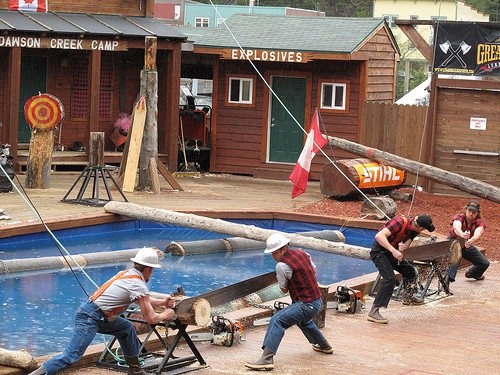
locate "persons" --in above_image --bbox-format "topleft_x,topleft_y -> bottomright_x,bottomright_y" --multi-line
245,233 -> 334,370
26,247 -> 175,375
367,214 -> 436,323
440,200 -> 490,295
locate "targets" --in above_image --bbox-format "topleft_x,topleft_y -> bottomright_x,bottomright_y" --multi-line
23,93 -> 65,131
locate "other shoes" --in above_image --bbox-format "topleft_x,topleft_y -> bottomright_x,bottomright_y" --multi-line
440,275 -> 450,292
464,268 -> 485,280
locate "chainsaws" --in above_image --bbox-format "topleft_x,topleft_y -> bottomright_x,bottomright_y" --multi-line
327,285 -> 365,314
188,314 -> 244,348
253,300 -> 290,326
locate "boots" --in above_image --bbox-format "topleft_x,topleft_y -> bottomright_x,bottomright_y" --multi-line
124,356 -> 157,375
402,289 -> 424,305
312,338 -> 333,354
244,346 -> 276,370
367,303 -> 389,324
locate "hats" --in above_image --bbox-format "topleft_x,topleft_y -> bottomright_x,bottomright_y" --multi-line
466,199 -> 480,212
416,214 -> 436,232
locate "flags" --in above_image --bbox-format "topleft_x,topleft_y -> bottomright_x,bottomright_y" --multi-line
289,110 -> 328,199
9,0 -> 49,14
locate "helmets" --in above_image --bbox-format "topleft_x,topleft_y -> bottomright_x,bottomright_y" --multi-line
130,247 -> 161,269
264,233 -> 291,254
